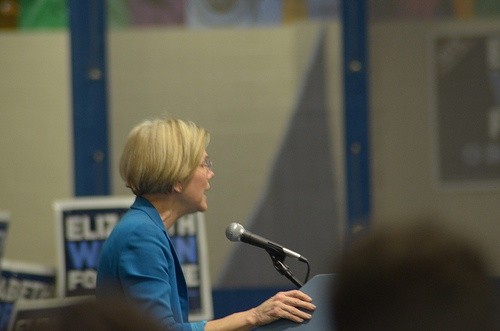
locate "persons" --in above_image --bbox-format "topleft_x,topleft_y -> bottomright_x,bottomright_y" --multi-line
94,117 -> 317,331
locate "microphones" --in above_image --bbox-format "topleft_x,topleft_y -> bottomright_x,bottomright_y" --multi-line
225,223 -> 308,262
